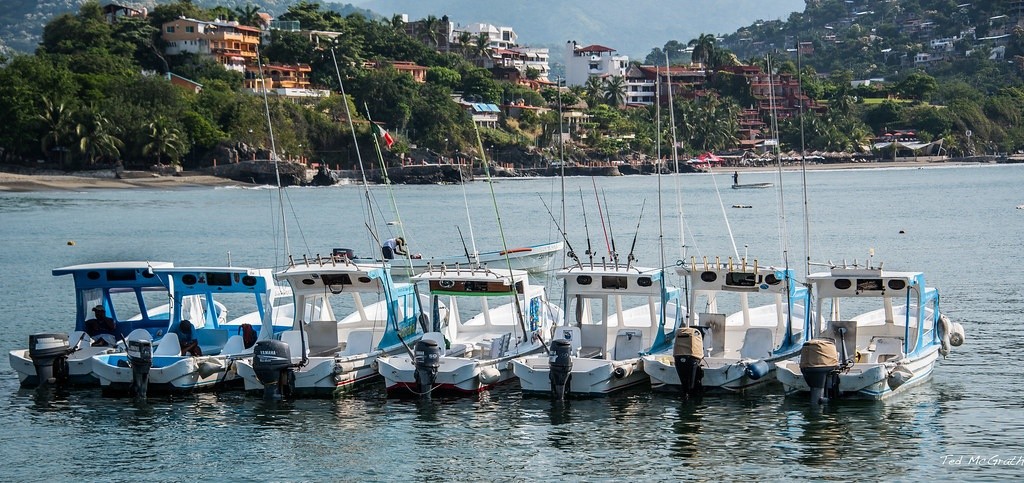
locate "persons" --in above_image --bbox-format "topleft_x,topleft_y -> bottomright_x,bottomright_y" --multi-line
731,171 -> 739,187
167,320 -> 201,357
88,305 -> 115,345
382,237 -> 406,260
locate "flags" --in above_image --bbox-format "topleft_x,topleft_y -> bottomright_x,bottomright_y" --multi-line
370,120 -> 394,149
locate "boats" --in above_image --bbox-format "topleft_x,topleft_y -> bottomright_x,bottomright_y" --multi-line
731,183 -> 774,189
642,260 -> 827,393
511,263 -> 699,395
377,260 -> 564,395
774,266 -> 967,405
7,264 -> 226,386
233,251 -> 449,390
92,264 -> 321,388
